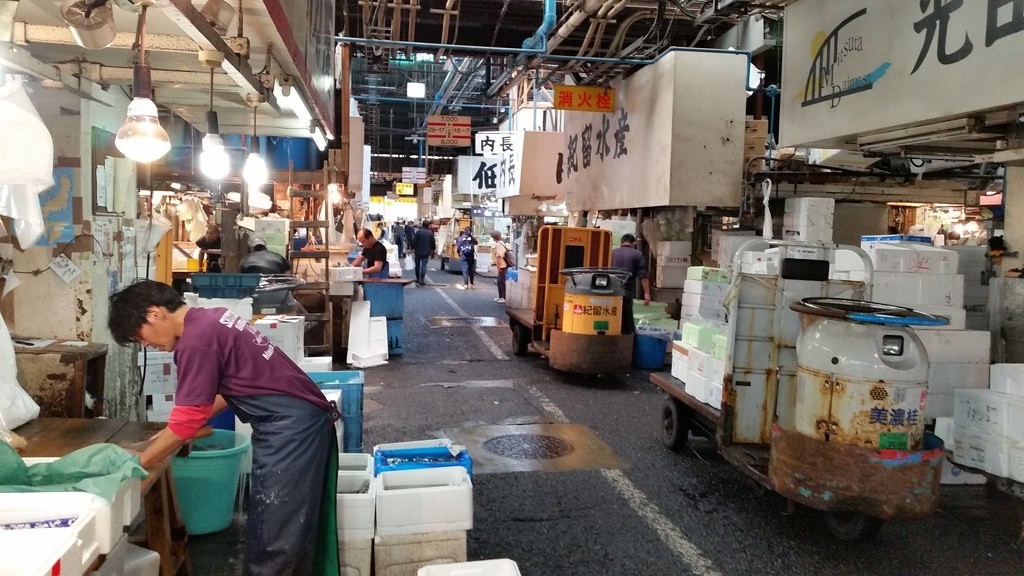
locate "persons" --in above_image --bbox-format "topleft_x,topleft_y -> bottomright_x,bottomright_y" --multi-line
394,220 -> 415,258
107,280 -> 342,576
196,225 -> 222,273
457,227 -> 478,289
490,230 -> 508,303
378,223 -> 386,238
239,244 -> 290,274
413,222 -> 436,285
351,229 -> 389,278
612,233 -> 650,335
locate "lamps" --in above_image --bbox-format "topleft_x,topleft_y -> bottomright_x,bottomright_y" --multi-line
59,0 -> 332,189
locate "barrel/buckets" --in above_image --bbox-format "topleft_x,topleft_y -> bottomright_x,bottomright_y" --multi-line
170,429 -> 250,534
634,325 -> 671,370
796,315 -> 930,448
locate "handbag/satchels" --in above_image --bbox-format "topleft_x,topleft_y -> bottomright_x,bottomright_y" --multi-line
503,250 -> 515,267
404,253 -> 415,270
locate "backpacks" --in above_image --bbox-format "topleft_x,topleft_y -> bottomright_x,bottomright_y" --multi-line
460,234 -> 473,254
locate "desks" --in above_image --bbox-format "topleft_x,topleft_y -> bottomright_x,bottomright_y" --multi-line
7,335 -> 109,418
0,415 -> 190,576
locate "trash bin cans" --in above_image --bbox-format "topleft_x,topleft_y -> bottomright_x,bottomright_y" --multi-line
633,325 -> 670,370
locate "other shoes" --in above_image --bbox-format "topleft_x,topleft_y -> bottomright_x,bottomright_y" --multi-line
415,279 -> 420,283
420,281 -> 425,285
470,283 -> 474,288
494,296 -> 506,303
464,284 -> 468,289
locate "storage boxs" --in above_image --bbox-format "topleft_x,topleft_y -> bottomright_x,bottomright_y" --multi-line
445,243 -> 462,258
382,243 -> 398,263
743,120 -> 768,174
599,197 -> 1024,486
459,219 -> 472,232
253,217 -> 290,263
775,144 -> 882,177
505,253 -> 537,311
0,268 -> 522,576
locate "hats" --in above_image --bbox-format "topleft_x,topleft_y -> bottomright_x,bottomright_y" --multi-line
489,230 -> 501,240
